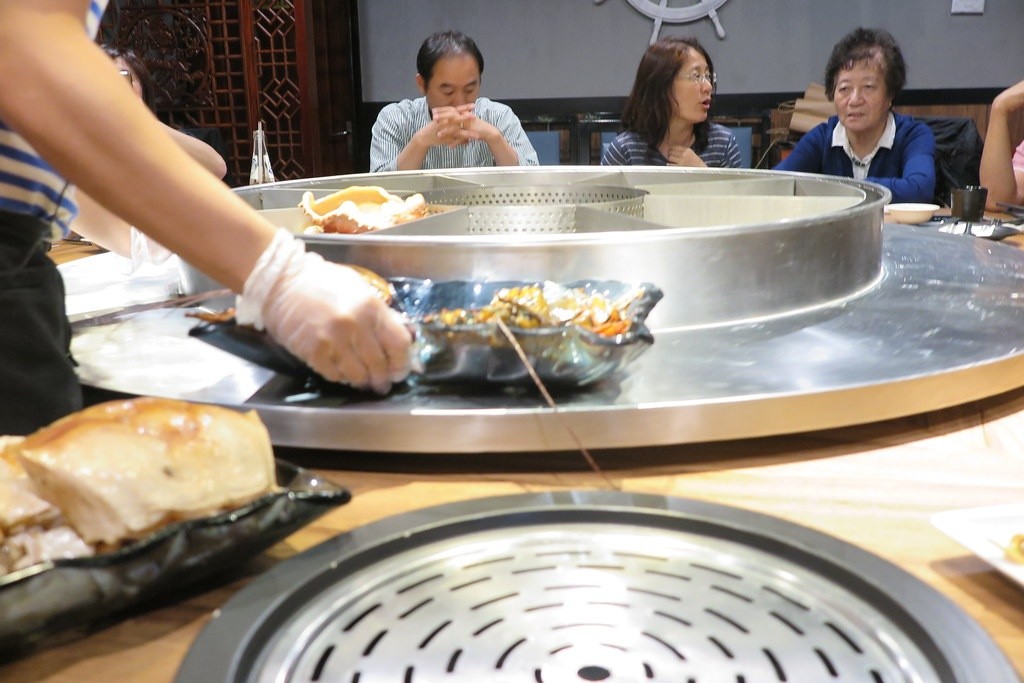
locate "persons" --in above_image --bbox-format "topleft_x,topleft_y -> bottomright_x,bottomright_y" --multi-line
98,43 -> 226,181
770,25 -> 936,203
369,30 -> 538,174
1,0 -> 416,441
599,34 -> 743,168
980,79 -> 1024,211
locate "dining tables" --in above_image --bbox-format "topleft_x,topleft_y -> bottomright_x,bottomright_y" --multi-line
0,168 -> 1024,683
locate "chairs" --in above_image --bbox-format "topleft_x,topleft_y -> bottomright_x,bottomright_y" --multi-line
521,120 -> 570,166
577,116 -> 984,209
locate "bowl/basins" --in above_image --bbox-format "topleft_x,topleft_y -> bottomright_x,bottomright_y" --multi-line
886,203 -> 940,223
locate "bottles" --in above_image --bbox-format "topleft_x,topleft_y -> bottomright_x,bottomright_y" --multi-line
249,130 -> 275,185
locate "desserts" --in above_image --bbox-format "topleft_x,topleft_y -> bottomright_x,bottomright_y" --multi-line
0,398 -> 277,573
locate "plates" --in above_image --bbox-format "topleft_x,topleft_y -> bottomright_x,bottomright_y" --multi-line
929,505 -> 1024,590
189,276 -> 663,386
1,457 -> 350,667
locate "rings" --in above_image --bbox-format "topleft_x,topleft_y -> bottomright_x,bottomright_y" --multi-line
461,122 -> 464,128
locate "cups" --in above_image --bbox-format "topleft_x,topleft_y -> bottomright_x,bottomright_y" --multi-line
950,185 -> 988,225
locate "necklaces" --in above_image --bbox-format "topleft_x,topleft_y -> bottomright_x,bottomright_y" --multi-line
850,143 -> 879,166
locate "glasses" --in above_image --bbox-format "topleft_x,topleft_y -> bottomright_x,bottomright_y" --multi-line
677,72 -> 717,82
